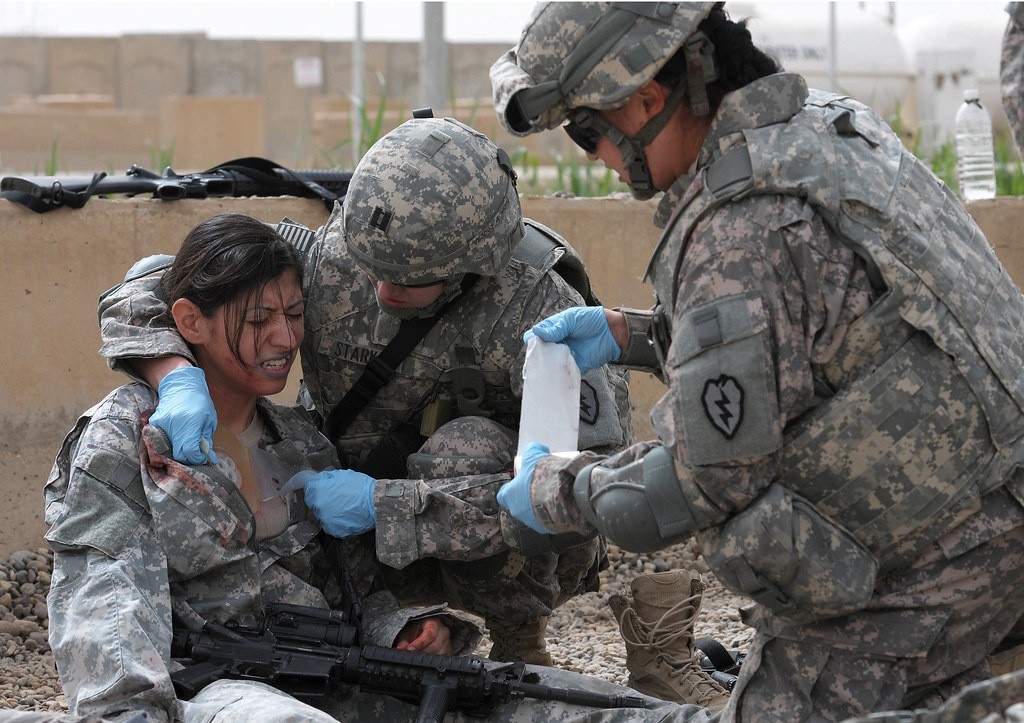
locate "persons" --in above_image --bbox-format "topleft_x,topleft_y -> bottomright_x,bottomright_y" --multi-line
46,213 -> 722,723
496,1 -> 1024,723
1000,0 -> 1024,143
95,106 -> 636,671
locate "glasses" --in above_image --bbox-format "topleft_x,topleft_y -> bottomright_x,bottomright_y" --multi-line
560,116 -> 604,155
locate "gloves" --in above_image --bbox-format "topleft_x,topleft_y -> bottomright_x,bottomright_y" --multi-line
304,469 -> 378,542
496,442 -> 562,535
525,307 -> 621,382
147,366 -> 220,468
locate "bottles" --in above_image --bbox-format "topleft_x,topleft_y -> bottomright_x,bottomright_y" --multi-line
956,89 -> 995,199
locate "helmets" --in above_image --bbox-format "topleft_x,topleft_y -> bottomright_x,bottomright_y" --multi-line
491,1 -> 728,138
341,107 -> 525,286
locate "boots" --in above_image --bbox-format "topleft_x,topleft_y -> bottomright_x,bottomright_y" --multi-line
487,617 -> 556,672
608,569 -> 735,713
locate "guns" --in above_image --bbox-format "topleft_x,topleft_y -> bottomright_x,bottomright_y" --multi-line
0,168 -> 354,201
169,600 -> 656,723
